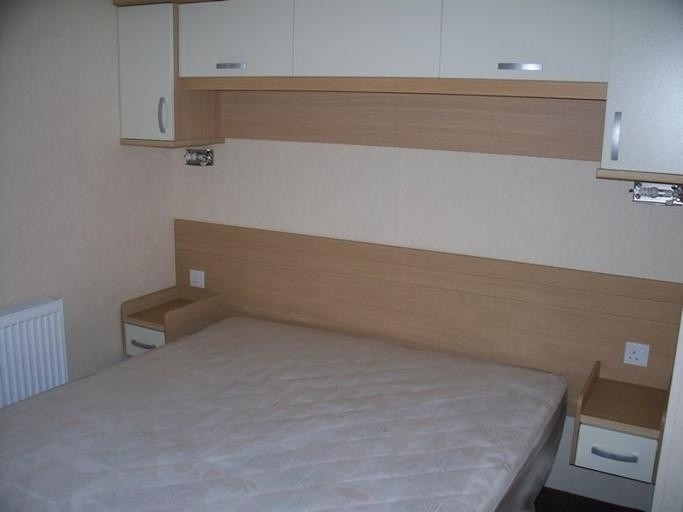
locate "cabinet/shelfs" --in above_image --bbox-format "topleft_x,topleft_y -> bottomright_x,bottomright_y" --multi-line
600,0 -> 682,174
114,0 -> 174,144
293,1 -> 443,81
120,321 -> 164,358
439,0 -> 607,83
176,1 -> 293,78
570,421 -> 658,484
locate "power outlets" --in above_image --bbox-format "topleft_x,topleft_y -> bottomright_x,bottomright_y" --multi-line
620,341 -> 651,367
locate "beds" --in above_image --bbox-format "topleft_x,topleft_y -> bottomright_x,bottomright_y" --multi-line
0,314 -> 567,510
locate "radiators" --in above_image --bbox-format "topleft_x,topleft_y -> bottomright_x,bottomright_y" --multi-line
1,298 -> 68,404
